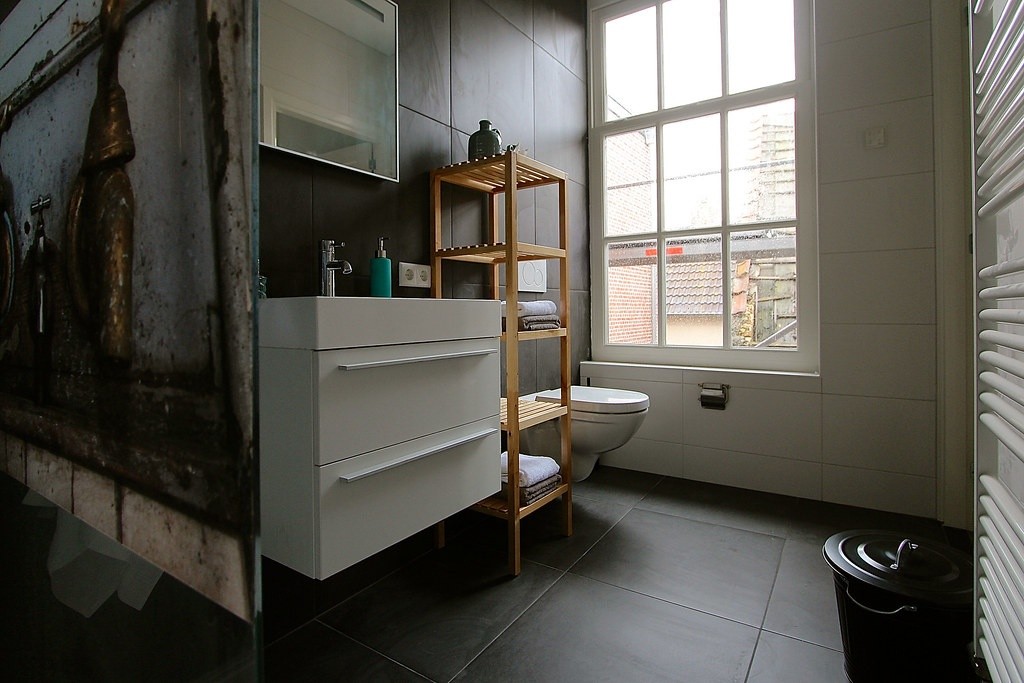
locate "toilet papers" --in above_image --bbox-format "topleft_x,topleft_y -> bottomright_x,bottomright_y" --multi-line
701,388 -> 726,399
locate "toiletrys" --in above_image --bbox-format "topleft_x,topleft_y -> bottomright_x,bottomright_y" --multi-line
369,236 -> 393,297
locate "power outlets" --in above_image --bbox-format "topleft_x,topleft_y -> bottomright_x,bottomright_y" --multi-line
399,262 -> 431,288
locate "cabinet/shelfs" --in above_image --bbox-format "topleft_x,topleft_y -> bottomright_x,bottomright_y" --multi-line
255,336 -> 502,583
429,149 -> 573,578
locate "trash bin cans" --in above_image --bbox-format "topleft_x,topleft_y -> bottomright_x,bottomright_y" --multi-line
821,529 -> 973,683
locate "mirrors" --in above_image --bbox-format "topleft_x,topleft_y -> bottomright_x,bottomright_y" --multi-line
253,0 -> 401,183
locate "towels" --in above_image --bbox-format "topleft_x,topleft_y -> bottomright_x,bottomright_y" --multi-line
501,300 -> 561,330
492,449 -> 563,506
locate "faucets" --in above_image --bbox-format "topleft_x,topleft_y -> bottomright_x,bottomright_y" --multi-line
320,239 -> 352,296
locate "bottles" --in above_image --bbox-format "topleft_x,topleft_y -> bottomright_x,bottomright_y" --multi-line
468,119 -> 502,162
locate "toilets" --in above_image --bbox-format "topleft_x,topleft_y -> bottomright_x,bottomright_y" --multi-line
518,386 -> 650,482
